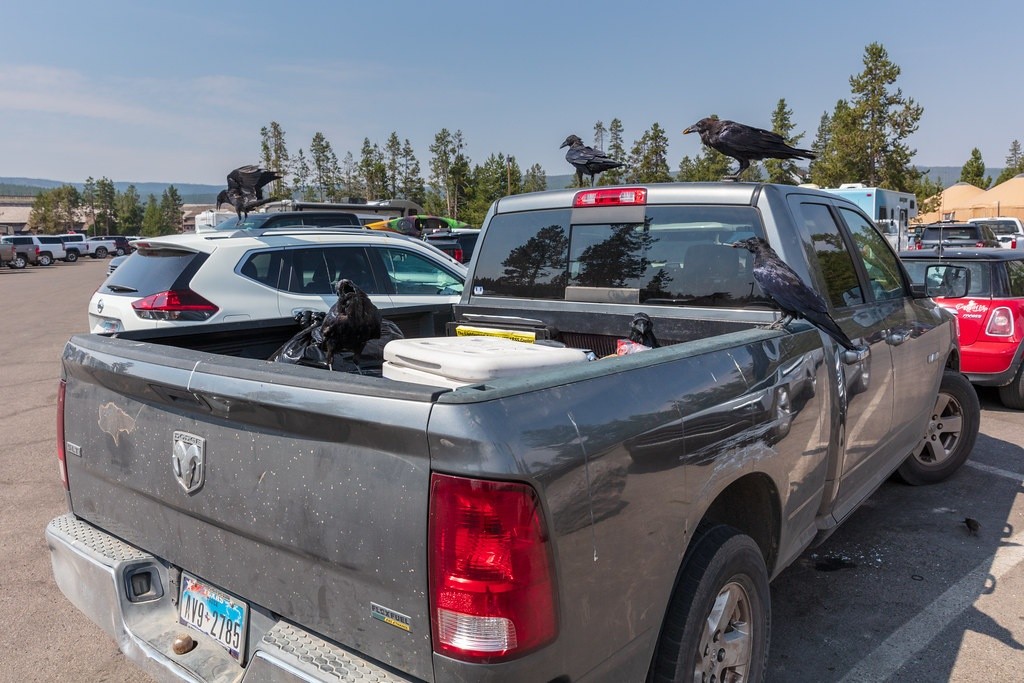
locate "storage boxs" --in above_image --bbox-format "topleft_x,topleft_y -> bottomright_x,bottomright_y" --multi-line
383,334 -> 586,391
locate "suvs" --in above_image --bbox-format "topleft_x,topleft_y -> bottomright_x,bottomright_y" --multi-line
88,195 -> 484,333
2,233 -> 150,266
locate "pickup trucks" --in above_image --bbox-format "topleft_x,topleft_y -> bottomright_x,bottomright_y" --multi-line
0,236 -> 40,269
45,181 -> 980,683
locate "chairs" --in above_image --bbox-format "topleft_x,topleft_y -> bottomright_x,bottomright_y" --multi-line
682,244 -> 739,302
303,258 -> 337,298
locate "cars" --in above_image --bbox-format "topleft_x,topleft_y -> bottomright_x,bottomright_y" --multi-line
875,216 -> 1024,410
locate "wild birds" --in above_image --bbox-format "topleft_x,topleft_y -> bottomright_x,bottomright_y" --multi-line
215,165 -> 282,227
733,237 -> 858,351
962,517 -> 982,537
311,279 -> 383,376
628,312 -> 662,348
683,118 -> 819,182
559,134 -> 626,187
